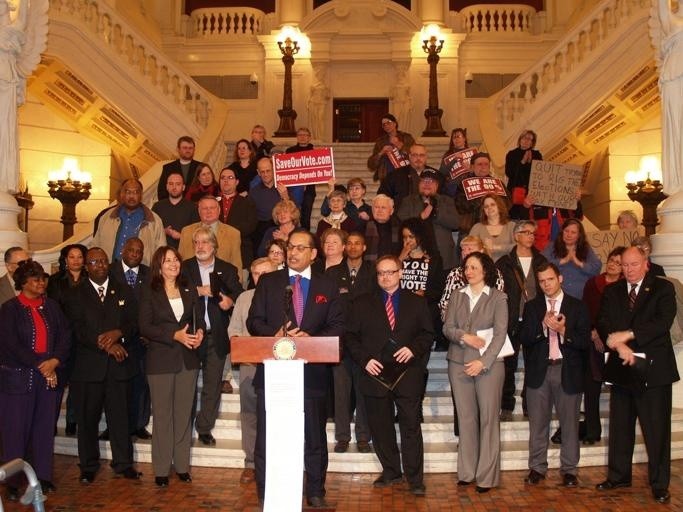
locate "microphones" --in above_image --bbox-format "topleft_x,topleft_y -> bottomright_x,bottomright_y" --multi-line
282,285 -> 293,314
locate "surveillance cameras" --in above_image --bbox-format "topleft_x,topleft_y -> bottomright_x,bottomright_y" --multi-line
250,73 -> 259,88
465,72 -> 474,85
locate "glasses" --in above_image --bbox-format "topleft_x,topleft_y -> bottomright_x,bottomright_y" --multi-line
288,244 -> 312,252
381,121 -> 393,125
518,230 -> 537,237
608,259 -> 622,267
376,269 -> 399,276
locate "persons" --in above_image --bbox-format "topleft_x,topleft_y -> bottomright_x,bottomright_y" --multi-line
317,115 -> 598,493
226,218 -> 443,511
1,227 -> 245,486
389,70 -> 415,134
306,68 -> 331,141
92,126 -> 317,394
584,211 -> 680,504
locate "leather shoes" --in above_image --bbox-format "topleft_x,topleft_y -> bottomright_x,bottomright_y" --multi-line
78,470 -> 93,482
654,489 -> 671,502
39,480 -> 56,495
564,474 -> 577,486
115,468 -> 139,478
357,441 -> 370,453
408,481 -> 425,495
334,441 -> 349,452
156,478 -> 167,485
198,432 -> 216,444
177,473 -> 191,481
596,479 -> 631,489
240,469 -> 256,484
221,381 -> 233,393
65,423 -> 76,433
374,473 -> 402,488
134,427 -> 151,440
524,470 -> 545,482
307,496 -> 328,507
101,429 -> 109,439
457,481 -> 469,486
4,487 -> 21,501
476,486 -> 491,491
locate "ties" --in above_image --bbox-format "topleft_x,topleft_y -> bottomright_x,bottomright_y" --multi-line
548,300 -> 560,361
385,293 -> 395,331
127,269 -> 135,289
292,275 -> 303,328
98,288 -> 105,306
627,284 -> 638,313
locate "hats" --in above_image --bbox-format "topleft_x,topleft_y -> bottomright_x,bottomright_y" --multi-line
14,262 -> 48,278
420,169 -> 438,179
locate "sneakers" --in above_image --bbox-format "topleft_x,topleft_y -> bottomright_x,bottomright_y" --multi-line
502,409 -> 513,420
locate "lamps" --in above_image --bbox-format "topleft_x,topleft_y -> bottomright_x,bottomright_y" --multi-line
465,72 -> 473,84
249,72 -> 258,85
